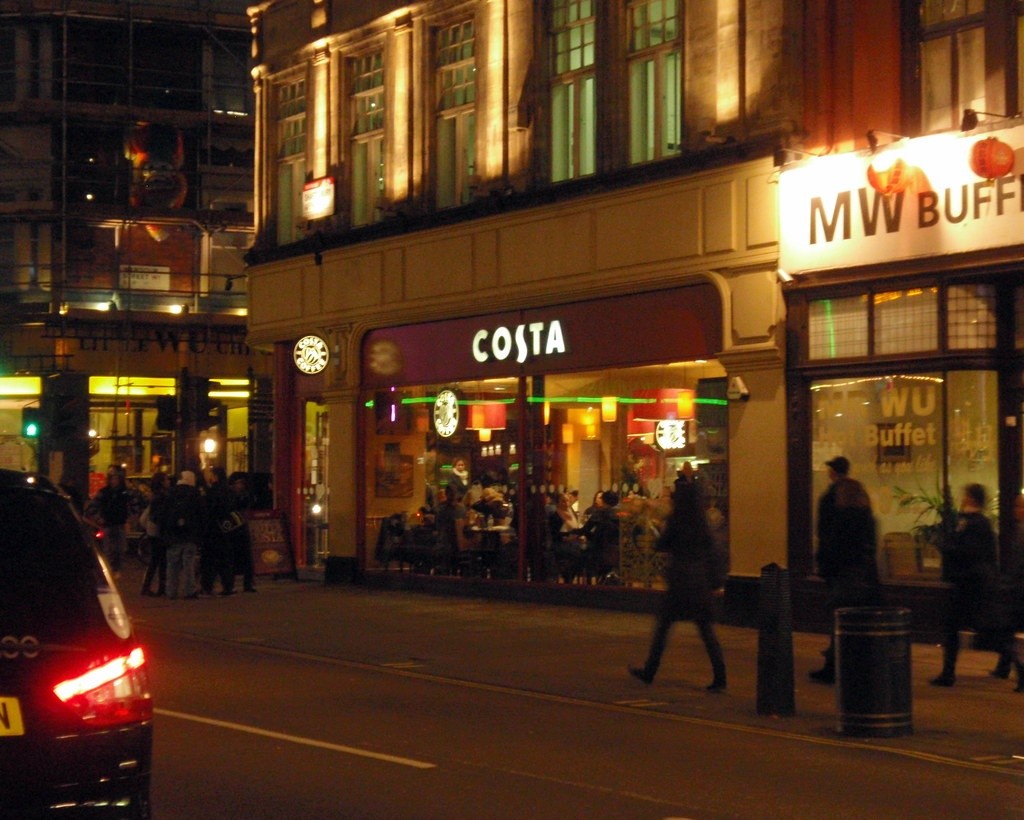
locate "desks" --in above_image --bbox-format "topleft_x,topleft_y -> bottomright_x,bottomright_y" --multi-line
472,524 -> 512,549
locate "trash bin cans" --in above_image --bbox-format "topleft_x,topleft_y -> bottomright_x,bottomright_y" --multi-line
835,605 -> 914,736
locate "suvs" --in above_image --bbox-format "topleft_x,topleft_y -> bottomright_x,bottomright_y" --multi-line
0,468 -> 160,820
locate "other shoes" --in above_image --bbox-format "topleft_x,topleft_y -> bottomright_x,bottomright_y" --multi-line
631,668 -> 654,684
1013,669 -> 1024,694
930,674 -> 954,686
708,680 -> 727,690
990,668 -> 1009,679
807,668 -> 836,683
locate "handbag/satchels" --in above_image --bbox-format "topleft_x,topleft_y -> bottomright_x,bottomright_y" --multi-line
139,504 -> 150,528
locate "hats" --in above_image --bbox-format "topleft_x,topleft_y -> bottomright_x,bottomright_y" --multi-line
177,471 -> 195,486
826,458 -> 848,474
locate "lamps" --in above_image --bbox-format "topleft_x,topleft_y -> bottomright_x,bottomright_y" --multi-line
315,248 -> 330,265
867,129 -> 905,154
226,275 -> 247,290
466,381 -> 694,444
773,145 -> 818,166
960,109 -> 1019,131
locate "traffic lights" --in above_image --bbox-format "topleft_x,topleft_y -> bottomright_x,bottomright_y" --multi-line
197,377 -> 223,432
23,409 -> 41,438
157,396 -> 176,429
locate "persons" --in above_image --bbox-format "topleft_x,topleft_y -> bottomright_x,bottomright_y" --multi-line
432,456 -> 719,586
810,456 -> 1024,693
59,463 -> 261,600
623,485 -> 732,693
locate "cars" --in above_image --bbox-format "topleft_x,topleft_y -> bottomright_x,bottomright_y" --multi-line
125,475 -> 153,538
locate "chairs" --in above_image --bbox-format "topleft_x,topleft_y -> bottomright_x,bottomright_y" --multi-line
376,516 -> 620,586
